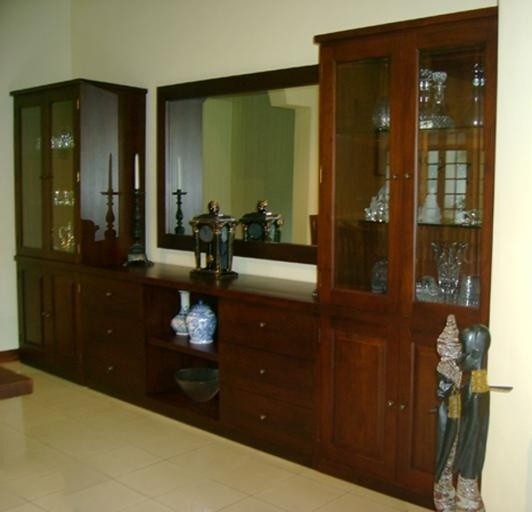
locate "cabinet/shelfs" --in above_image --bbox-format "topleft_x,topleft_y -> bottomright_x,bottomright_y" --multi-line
77,263 -> 149,404
147,280 -> 222,432
312,6 -> 498,311
221,292 -> 325,481
9,77 -> 146,263
323,304 -> 485,512
14,254 -> 78,389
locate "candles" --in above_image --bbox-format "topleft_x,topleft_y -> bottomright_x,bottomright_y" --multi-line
176,155 -> 181,190
134,152 -> 140,191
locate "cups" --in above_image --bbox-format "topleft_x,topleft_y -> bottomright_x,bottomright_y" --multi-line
458,274 -> 481,307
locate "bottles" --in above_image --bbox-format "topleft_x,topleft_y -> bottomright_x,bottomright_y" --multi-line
419,64 -> 486,130
371,255 -> 388,293
170,291 -> 216,344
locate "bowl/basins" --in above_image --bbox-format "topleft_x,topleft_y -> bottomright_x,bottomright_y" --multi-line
174,367 -> 219,403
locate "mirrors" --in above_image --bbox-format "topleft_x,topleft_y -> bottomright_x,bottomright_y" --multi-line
156,64 -> 319,266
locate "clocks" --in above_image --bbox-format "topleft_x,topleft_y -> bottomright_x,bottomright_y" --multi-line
188,200 -> 238,282
238,200 -> 282,243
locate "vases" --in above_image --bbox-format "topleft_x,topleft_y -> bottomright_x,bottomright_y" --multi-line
428,241 -> 471,304
186,299 -> 217,345
170,290 -> 193,336
